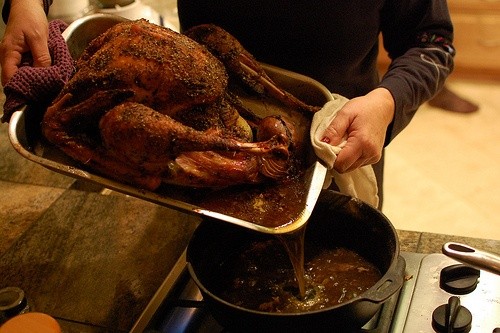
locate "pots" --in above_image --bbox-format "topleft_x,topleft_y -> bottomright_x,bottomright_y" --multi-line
186,190 -> 407,333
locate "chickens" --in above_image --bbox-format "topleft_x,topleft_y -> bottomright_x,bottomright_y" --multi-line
44,20 -> 324,191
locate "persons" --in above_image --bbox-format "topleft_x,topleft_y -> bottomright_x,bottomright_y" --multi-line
0,0 -> 459,215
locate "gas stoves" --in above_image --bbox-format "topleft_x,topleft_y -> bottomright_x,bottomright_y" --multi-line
156,250 -> 500,333
